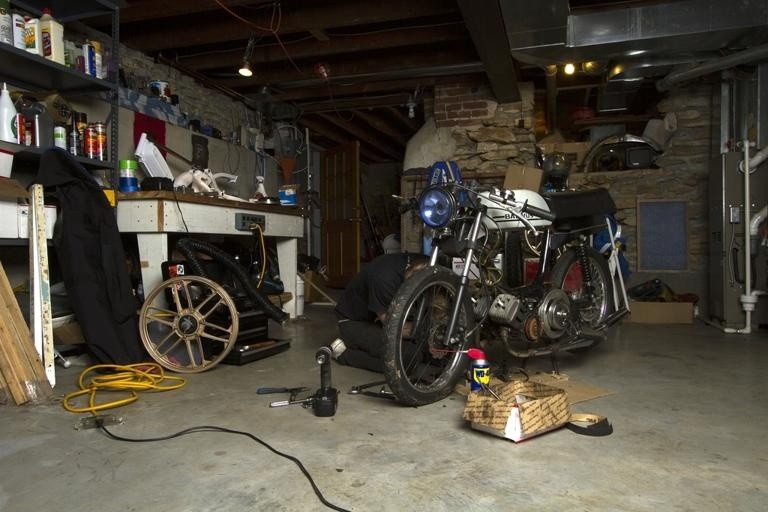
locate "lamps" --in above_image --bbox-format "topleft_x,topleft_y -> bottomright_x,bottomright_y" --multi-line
236,40 -> 256,78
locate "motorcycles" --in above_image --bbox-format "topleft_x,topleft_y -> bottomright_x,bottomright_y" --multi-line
382,184 -> 630,406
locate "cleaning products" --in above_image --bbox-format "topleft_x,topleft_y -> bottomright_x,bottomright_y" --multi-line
0,0 -> 103,79
0,80 -> 107,162
254,176 -> 268,198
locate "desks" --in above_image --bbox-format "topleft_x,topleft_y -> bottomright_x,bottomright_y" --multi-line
117,190 -> 305,319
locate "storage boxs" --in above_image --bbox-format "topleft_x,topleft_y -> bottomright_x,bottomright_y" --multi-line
299,270 -> 329,304
462,380 -> 574,443
502,164 -> 544,194
553,141 -> 592,168
624,299 -> 696,326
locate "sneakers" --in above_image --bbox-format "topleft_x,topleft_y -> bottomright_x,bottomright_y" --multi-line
328,337 -> 348,361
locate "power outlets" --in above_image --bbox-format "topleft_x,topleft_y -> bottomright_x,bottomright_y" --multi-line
235,212 -> 265,233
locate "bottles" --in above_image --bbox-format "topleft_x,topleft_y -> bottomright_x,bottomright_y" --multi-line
83,122 -> 99,160
69,110 -> 81,157
95,121 -> 109,162
118,159 -> 138,192
54,120 -> 67,151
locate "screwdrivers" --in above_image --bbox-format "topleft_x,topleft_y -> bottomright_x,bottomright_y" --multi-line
270,398 -> 312,407
257,387 -> 311,394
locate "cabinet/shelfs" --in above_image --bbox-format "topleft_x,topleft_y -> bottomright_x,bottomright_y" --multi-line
0,0 -> 121,170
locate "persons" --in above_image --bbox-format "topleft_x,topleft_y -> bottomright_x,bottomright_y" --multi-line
329,250 -> 448,376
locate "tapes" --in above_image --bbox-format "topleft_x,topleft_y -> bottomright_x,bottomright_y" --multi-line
119,160 -> 139,192
